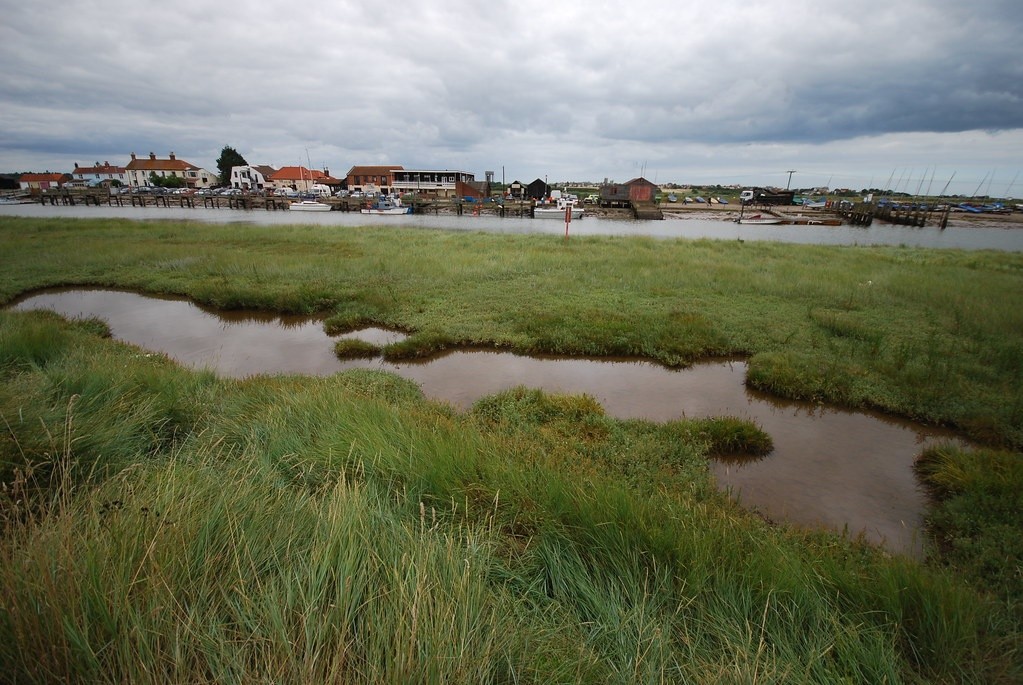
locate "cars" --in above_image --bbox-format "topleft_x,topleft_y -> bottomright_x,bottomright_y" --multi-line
119,183 -> 331,198
684,196 -> 694,203
667,193 -> 678,202
717,196 -> 728,204
711,197 -> 718,204
696,196 -> 705,203
584,194 -> 599,205
335,189 -> 415,198
655,193 -> 663,200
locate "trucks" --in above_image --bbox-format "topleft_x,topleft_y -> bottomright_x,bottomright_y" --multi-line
740,188 -> 795,206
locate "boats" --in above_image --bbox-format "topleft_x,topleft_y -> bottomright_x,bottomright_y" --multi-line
360,200 -> 409,215
533,197 -> 585,220
289,200 -> 332,212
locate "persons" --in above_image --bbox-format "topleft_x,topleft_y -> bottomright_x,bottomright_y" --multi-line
681,193 -> 687,205
707,196 -> 712,206
592,196 -> 597,204
